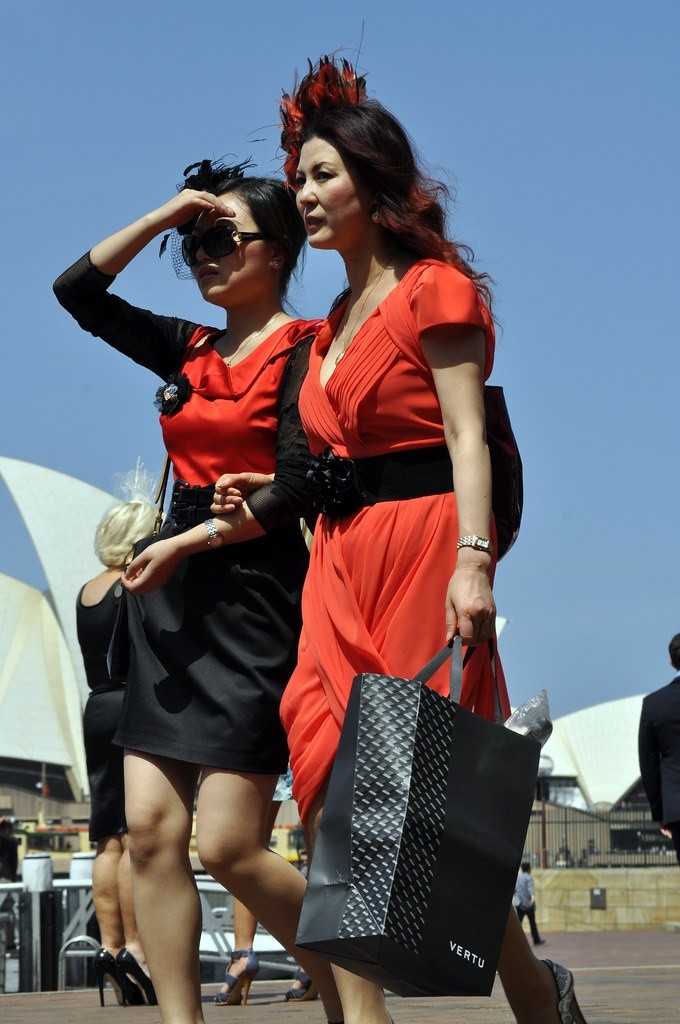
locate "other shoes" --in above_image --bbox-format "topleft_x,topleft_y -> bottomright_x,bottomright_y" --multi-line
535,940 -> 546,944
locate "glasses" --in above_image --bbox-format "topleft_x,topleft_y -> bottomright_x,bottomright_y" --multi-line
181,224 -> 265,267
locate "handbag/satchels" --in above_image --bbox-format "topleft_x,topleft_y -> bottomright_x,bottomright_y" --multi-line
294,627 -> 543,998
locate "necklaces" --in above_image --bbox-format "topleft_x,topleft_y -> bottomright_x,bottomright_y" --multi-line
225,311 -> 284,366
336,269 -> 386,363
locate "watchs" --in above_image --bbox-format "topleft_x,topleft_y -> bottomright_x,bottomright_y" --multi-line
456,536 -> 493,553
204,519 -> 224,547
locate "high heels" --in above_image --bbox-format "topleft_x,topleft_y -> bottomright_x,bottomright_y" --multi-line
542,956 -> 588,1024
214,949 -> 260,1006
285,965 -> 319,1001
93,947 -> 158,1008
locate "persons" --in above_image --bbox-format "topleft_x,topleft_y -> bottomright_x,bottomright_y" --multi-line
0,820 -> 17,960
212,61 -> 586,1024
515,862 -> 545,945
638,633 -> 680,867
54,161 -> 342,1024
213,761 -> 319,1005
77,503 -> 167,1008
